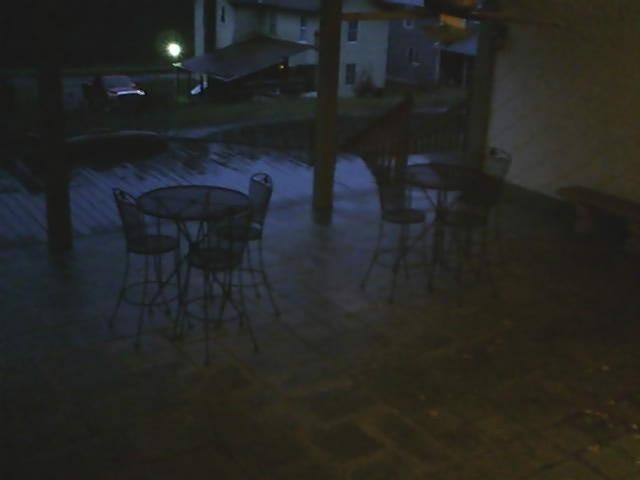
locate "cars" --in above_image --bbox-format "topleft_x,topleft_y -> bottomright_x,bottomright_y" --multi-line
99,73 -> 146,97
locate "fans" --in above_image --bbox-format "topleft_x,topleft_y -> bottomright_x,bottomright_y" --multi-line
264,1 -> 569,47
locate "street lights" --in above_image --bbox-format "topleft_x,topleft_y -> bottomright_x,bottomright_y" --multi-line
165,41 -> 183,110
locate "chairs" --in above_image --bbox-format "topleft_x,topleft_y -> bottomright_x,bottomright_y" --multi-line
109,171 -> 275,367
359,146 -> 514,303
